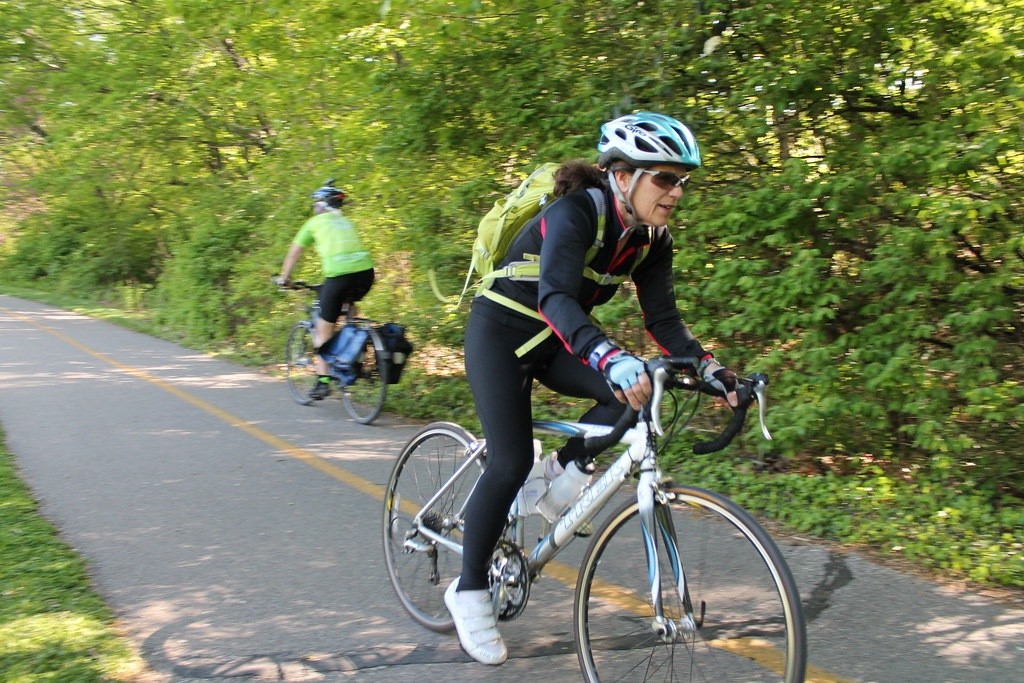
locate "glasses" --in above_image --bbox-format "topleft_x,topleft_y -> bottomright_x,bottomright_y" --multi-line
633,167 -> 691,192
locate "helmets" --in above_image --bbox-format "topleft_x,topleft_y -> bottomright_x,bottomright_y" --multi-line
598,110 -> 701,172
310,187 -> 346,209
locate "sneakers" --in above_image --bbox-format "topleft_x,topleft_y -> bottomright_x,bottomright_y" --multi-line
444,575 -> 508,666
541,451 -> 594,537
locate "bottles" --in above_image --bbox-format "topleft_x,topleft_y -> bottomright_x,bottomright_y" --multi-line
535,454 -> 595,524
524,439 -> 547,516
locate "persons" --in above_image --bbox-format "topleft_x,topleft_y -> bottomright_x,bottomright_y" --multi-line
443,110 -> 745,667
274,186 -> 375,397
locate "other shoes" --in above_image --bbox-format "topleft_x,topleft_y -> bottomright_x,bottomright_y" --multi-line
309,377 -> 331,397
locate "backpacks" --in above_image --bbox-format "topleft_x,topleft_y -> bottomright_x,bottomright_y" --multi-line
473,163 -> 654,298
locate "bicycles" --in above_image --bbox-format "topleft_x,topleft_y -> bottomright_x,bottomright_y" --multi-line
380,354 -> 808,682
271,273 -> 389,425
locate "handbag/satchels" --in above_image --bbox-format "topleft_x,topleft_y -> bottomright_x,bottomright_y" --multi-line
318,324 -> 369,388
375,322 -> 413,384
